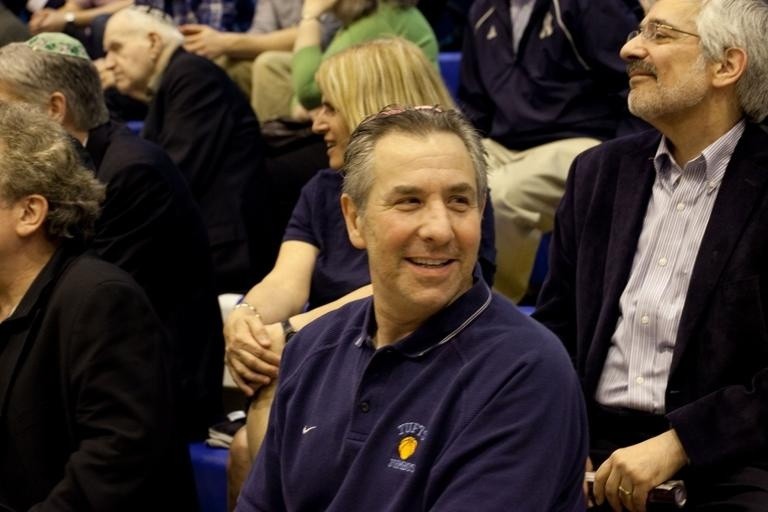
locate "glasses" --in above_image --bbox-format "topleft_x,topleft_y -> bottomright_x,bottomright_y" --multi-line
627,22 -> 699,39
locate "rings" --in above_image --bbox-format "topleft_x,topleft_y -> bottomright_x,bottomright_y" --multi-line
617,486 -> 632,496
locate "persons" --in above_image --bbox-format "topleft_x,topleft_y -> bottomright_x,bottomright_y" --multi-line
528,0 -> 766,510
232,103 -> 589,512
0,32 -> 225,443
0,104 -> 171,512
0,0 -> 645,308
219,31 -> 495,512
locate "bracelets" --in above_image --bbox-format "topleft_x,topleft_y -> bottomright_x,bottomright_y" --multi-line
280,318 -> 296,343
234,302 -> 262,320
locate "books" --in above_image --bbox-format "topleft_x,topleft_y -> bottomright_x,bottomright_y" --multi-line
205,410 -> 246,451
